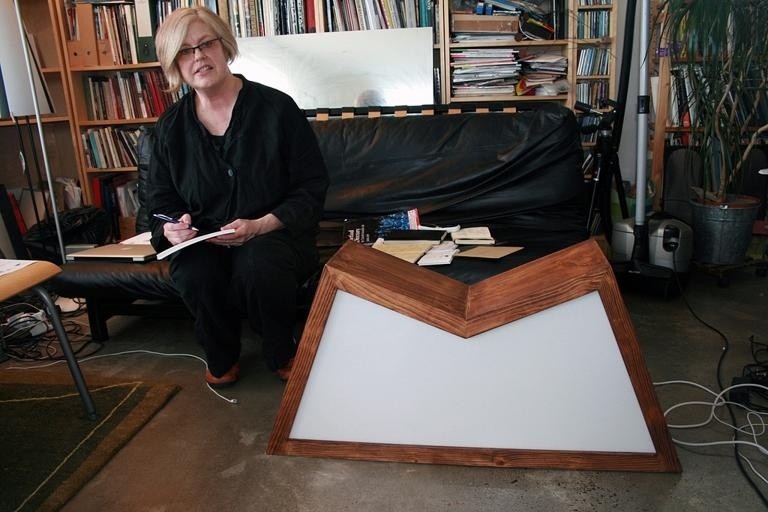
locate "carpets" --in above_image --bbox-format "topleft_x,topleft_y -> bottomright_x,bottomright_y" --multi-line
0,378 -> 178,512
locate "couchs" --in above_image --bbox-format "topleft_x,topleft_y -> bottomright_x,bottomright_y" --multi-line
59,108 -> 593,341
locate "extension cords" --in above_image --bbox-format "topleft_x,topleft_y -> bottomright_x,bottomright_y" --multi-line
6,312 -> 54,335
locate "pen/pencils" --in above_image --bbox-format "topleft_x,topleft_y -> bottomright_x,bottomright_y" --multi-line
153,213 -> 200,232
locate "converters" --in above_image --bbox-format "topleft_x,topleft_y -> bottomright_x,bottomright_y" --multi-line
730,377 -> 750,401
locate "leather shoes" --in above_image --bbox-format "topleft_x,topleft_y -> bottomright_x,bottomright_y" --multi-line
205,360 -> 240,387
274,359 -> 293,380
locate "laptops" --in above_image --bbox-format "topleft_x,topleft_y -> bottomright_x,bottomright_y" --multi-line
66,243 -> 157,264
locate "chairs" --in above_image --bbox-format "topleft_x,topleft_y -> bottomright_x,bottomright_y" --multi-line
1,259 -> 98,417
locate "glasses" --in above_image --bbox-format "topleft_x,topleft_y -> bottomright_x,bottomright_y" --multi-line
179,36 -> 224,56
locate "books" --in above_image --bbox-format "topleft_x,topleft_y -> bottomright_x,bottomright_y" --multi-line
575,0 -> 614,109
155,228 -> 235,262
454,226 -> 495,245
64,2 -> 442,216
417,248 -> 460,267
650,6 -> 768,155
384,228 -> 448,245
448,0 -> 567,99
371,242 -> 434,264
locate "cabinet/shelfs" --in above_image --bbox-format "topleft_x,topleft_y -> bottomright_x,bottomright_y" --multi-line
1,1 -> 87,211
655,0 -> 768,236
52,1 -> 618,198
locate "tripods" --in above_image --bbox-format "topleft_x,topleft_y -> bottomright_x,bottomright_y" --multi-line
574,97 -> 629,237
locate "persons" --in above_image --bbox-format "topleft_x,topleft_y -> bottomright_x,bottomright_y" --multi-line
145,6 -> 331,386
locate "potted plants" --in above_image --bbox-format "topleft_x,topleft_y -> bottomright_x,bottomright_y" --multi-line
638,0 -> 768,266
